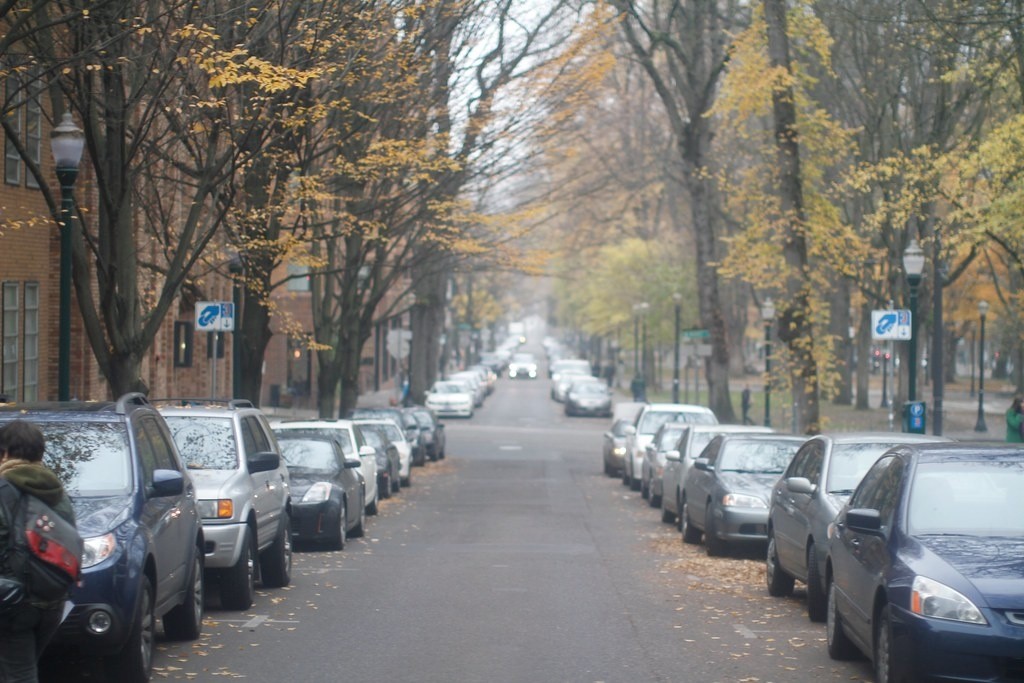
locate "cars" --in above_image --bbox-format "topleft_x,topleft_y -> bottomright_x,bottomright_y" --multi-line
265,406 -> 447,517
542,338 -> 614,418
422,328 -> 538,417
271,436 -> 370,554
825,442 -> 1024,683
678,435 -> 815,556
764,435 -> 958,621
601,402 -> 775,530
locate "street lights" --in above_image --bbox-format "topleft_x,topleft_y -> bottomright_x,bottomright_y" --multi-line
669,294 -> 686,407
48,112 -> 87,400
974,300 -> 990,432
631,301 -> 650,402
759,297 -> 777,427
902,237 -> 932,435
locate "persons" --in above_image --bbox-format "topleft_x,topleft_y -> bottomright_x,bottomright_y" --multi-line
0,420 -> 78,683
1005,394 -> 1024,444
741,384 -> 758,425
630,371 -> 646,403
591,359 -> 627,391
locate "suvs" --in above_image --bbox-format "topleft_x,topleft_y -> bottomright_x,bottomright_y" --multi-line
0,393 -> 207,683
146,396 -> 295,611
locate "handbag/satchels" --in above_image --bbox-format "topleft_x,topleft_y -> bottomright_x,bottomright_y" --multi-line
0,478 -> 84,601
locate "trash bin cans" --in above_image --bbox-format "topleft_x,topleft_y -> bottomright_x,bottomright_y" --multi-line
268,383 -> 281,408
901,401 -> 927,434
